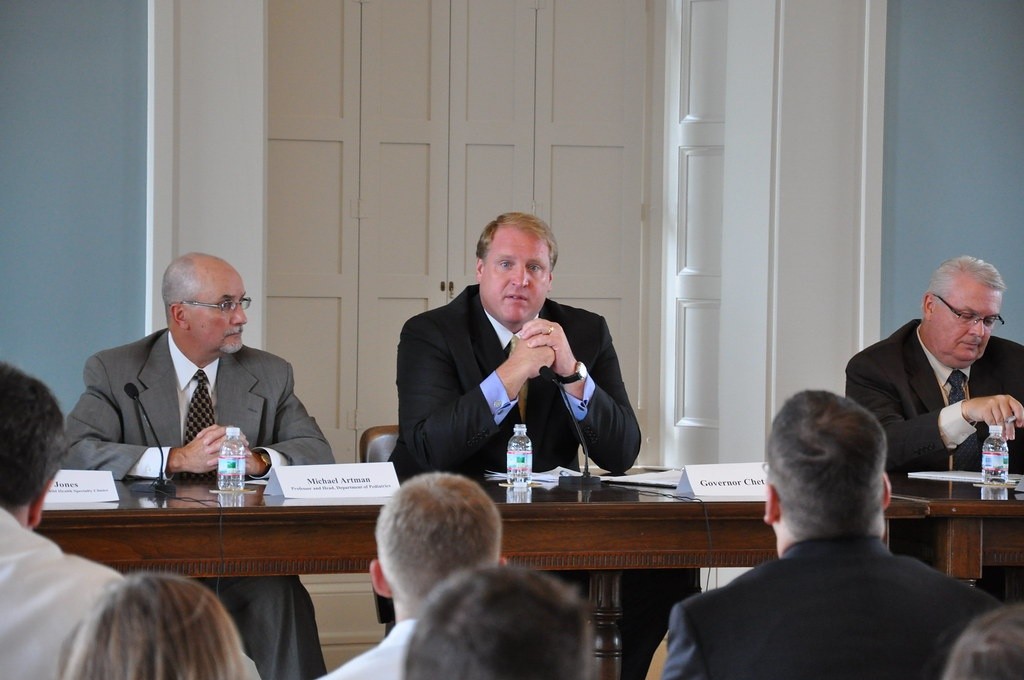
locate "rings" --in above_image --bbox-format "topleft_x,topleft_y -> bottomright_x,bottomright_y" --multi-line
547,325 -> 553,334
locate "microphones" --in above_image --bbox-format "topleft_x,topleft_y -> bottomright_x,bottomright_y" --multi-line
123,383 -> 177,494
538,365 -> 602,492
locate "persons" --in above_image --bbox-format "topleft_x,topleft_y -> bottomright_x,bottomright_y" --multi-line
0,363 -> 260,680
58,251 -> 336,680
659,389 -> 1024,680
316,470 -> 595,680
373,213 -> 702,680
845,256 -> 1024,602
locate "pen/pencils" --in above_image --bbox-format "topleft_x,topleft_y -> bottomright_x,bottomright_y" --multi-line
1006,417 -> 1016,424
558,470 -> 573,477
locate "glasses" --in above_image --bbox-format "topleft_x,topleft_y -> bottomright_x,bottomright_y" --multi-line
932,294 -> 1005,326
168,297 -> 251,311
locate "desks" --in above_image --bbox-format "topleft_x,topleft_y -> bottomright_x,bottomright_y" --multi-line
33,465 -> 1024,680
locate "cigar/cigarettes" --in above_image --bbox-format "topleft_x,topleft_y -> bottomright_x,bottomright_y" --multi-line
1004,415 -> 1016,423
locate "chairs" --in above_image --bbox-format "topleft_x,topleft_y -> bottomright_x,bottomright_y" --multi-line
360,425 -> 406,635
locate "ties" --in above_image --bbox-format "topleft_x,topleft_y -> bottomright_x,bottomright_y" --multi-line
947,370 -> 981,496
183,369 -> 215,482
511,334 -> 527,423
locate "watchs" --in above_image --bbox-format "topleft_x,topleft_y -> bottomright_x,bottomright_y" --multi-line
557,361 -> 587,385
256,451 -> 271,478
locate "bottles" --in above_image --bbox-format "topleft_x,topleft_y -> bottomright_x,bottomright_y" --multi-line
505,485 -> 531,503
506,423 -> 532,486
217,493 -> 244,507
218,426 -> 246,490
980,486 -> 1008,500
981,425 -> 1009,486
